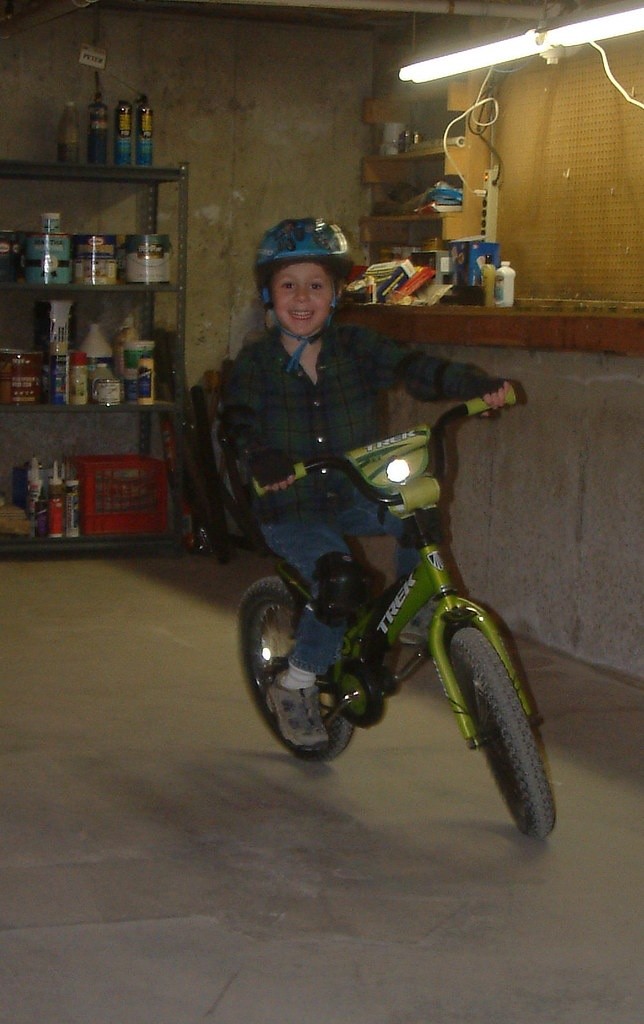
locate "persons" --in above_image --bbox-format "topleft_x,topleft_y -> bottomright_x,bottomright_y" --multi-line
217,218 -> 512,751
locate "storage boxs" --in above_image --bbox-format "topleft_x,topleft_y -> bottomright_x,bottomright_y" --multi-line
67,455 -> 167,536
449,242 -> 499,286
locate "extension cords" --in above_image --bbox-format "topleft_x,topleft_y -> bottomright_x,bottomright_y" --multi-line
481,168 -> 500,244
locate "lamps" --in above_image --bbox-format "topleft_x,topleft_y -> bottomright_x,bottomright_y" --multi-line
398,0 -> 644,85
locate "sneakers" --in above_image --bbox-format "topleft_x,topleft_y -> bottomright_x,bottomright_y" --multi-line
398,601 -> 439,645
266,670 -> 329,752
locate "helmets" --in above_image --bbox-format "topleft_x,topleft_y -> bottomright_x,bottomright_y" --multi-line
254,218 -> 353,303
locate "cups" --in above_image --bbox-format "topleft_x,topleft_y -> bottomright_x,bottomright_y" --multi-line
40,213 -> 63,234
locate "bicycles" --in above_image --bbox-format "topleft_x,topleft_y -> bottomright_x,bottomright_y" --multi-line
238,381 -> 557,840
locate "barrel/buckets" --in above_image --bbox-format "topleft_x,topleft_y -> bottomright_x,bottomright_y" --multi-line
125,233 -> 172,283
0,349 -> 44,406
0,229 -> 21,283
24,233 -> 118,285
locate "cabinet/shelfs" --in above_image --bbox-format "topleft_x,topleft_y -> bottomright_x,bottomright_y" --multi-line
359,37 -> 493,243
0,158 -> 190,560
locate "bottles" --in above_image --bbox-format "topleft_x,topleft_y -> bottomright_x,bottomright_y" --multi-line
48,493 -> 64,537
115,100 -> 132,166
390,139 -> 398,155
114,317 -> 140,402
413,131 -> 419,144
96,380 -> 121,406
124,340 -> 156,404
137,354 -> 155,404
70,352 -> 89,406
91,363 -> 115,403
495,261 -> 516,307
482,254 -> 495,308
58,101 -> 80,165
403,130 -> 411,152
34,487 -> 48,537
80,324 -> 114,401
65,480 -> 79,538
136,95 -> 153,168
30,478 -> 41,514
89,92 -> 107,167
47,462 -> 64,500
